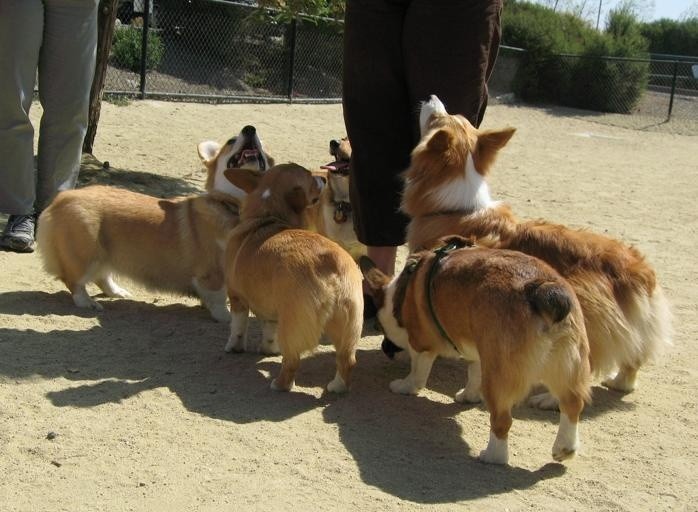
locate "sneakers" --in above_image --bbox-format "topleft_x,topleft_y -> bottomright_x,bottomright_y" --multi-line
0,215 -> 34,251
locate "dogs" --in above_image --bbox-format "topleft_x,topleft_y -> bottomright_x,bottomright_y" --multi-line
357,233 -> 596,466
393,93 -> 678,411
222,159 -> 365,396
34,125 -> 277,324
302,134 -> 370,264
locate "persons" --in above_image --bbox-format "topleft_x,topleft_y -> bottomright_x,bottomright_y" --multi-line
343,0 -> 505,321
0,0 -> 98,253
